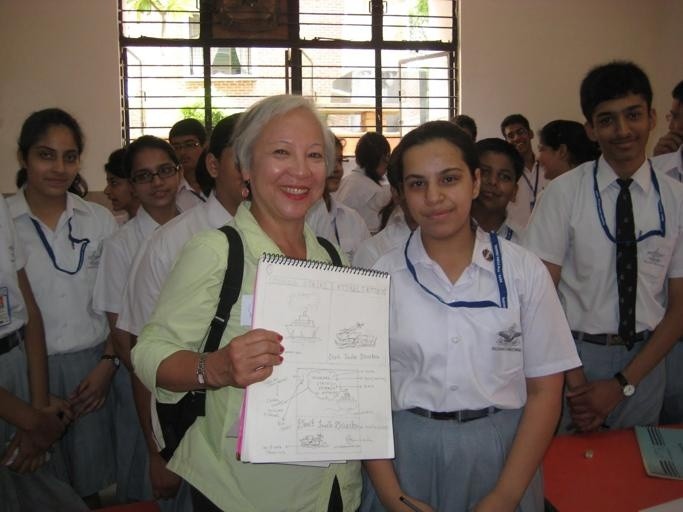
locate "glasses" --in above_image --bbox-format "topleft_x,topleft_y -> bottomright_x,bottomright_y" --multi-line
174,141 -> 201,152
131,165 -> 180,185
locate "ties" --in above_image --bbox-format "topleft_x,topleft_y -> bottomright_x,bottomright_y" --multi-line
616,179 -> 637,352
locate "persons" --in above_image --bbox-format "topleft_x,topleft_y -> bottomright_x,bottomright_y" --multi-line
1,62 -> 683,511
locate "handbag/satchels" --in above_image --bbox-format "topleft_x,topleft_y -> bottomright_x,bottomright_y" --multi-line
150,391 -> 204,464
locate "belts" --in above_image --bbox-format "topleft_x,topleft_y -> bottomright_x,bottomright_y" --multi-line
573,330 -> 650,347
407,407 -> 499,423
2,327 -> 25,357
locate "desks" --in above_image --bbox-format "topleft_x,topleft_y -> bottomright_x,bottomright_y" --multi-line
532,423 -> 681,512
